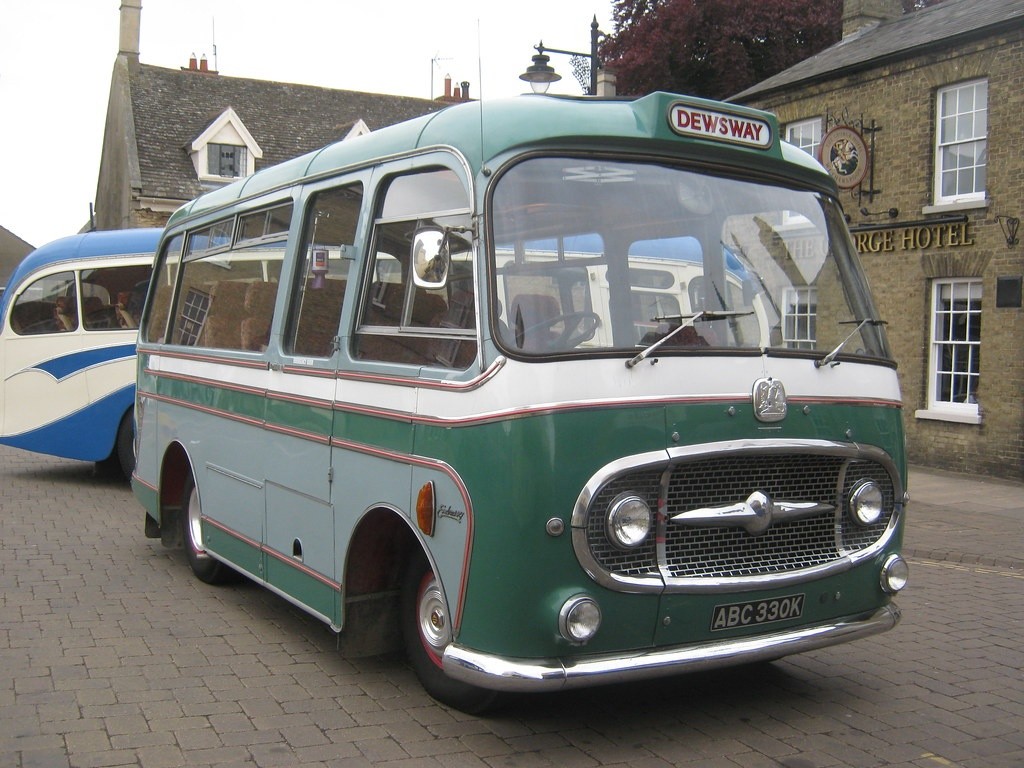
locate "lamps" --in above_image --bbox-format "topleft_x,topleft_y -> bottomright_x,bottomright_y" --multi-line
860,207 -> 898,218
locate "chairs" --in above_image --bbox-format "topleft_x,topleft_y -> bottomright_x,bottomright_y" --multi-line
510,295 -> 565,348
7,286 -> 144,336
147,282 -> 475,372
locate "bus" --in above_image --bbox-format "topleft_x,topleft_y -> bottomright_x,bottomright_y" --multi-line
1,225 -> 779,488
126,85 -> 917,718
